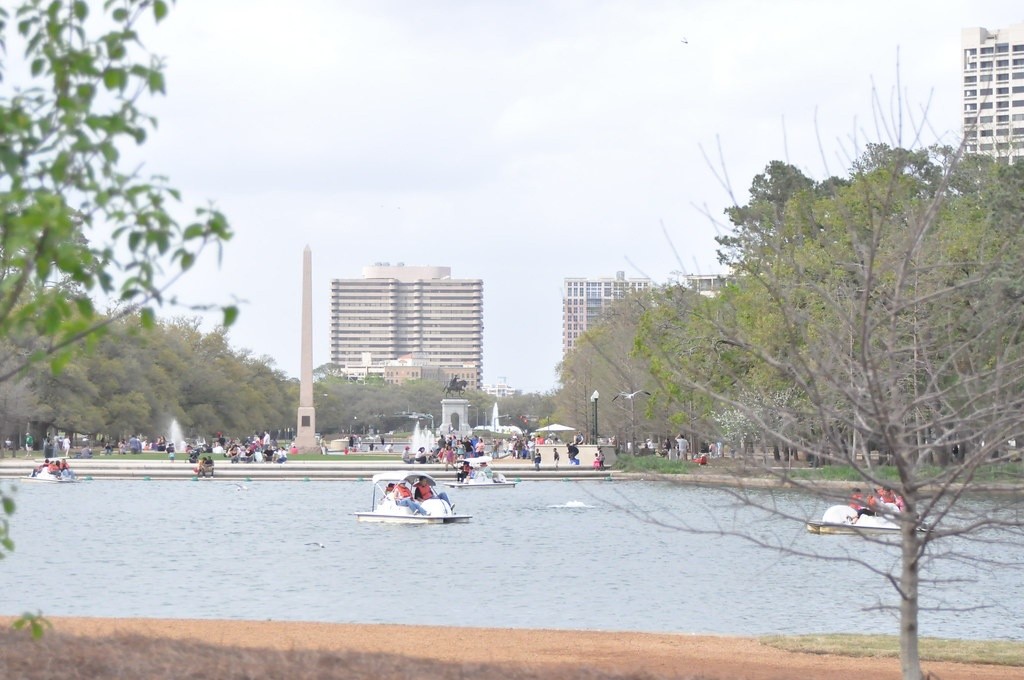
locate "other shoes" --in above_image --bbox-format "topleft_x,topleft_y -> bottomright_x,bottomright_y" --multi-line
414,509 -> 418,514
426,512 -> 430,516
450,504 -> 455,510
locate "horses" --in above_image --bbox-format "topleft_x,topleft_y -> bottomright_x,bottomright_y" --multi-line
442,380 -> 467,397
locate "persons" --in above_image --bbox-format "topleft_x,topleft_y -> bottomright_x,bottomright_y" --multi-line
166,443 -> 214,475
846,486 -> 904,524
385,476 -> 455,516
43,432 -> 93,459
504,434 -> 536,464
32,458 -> 74,481
101,433 -> 166,456
400,434 -> 499,484
450,375 -> 460,391
709,440 -> 722,459
534,432 -> 584,471
594,447 -> 605,471
5,437 -> 11,452
662,434 -> 700,460
320,434 -> 394,455
214,430 -> 297,464
25,433 -> 33,457
644,439 -> 653,448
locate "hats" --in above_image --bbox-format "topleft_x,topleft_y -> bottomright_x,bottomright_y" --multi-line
405,446 -> 410,450
398,480 -> 405,484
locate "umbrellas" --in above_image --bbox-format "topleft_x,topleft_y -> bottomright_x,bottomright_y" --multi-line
535,424 -> 576,445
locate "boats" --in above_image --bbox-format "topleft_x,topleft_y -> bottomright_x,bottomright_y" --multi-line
19,466 -> 78,483
444,467 -> 524,491
804,505 -> 935,535
352,471 -> 473,527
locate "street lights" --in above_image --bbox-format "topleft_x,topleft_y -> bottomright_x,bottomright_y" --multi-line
354,416 -> 357,434
468,404 -> 480,428
546,415 -> 550,438
591,390 -> 598,444
589,396 -> 595,448
